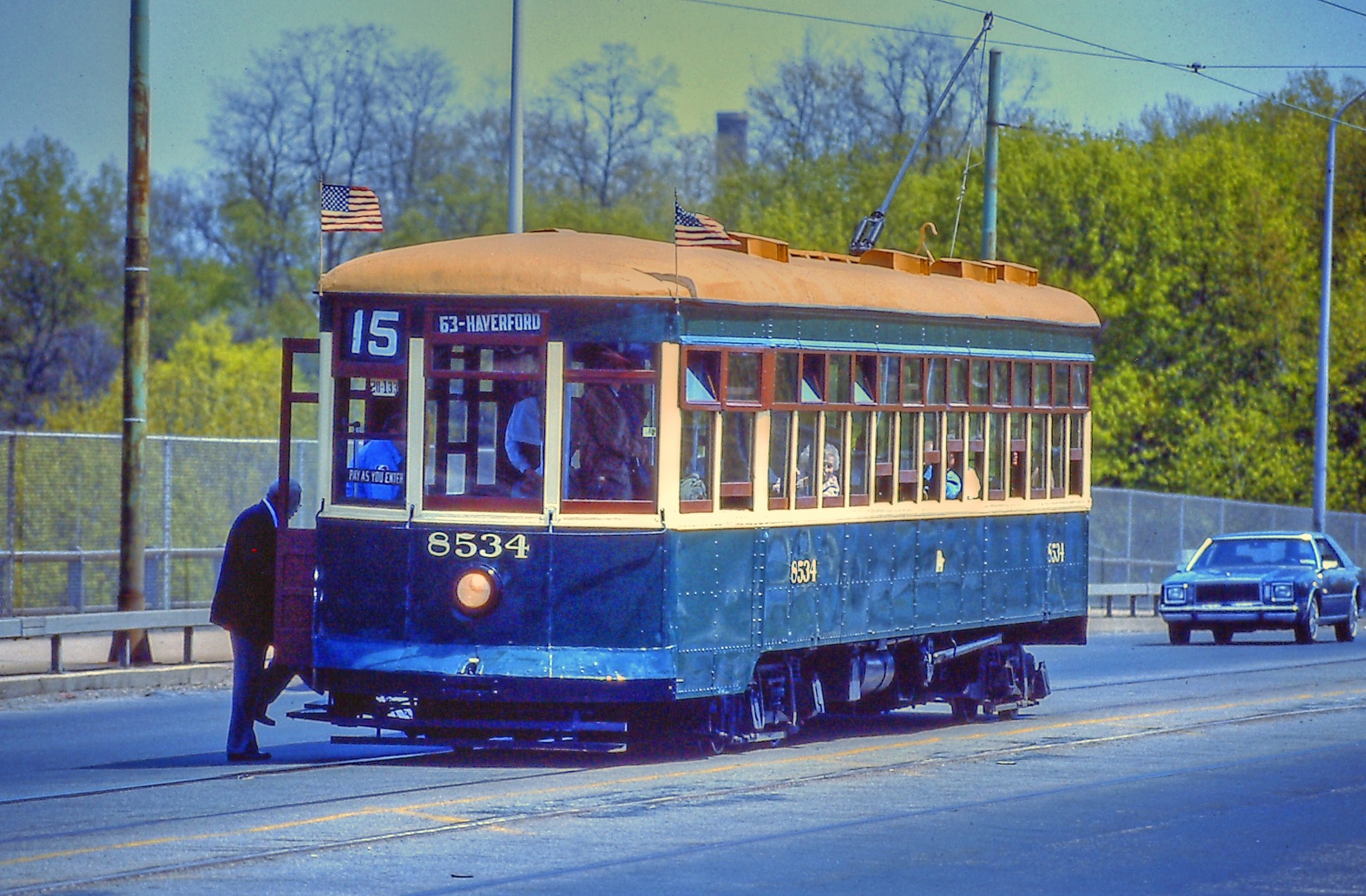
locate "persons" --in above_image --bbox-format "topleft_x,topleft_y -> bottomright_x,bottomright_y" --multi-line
680,457 -> 709,498
963,464 -> 981,500
796,439 -> 840,496
210,478 -> 301,760
579,352 -> 647,500
1012,448 -> 1053,492
346,412 -> 408,504
922,453 -> 961,499
504,355 -> 586,500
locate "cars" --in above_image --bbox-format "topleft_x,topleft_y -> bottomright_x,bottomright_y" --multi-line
1159,529 -> 1366,646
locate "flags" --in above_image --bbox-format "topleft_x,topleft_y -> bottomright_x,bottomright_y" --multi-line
674,201 -> 743,247
320,183 -> 386,232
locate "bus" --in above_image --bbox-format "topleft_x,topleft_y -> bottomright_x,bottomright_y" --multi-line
275,229 -> 1100,755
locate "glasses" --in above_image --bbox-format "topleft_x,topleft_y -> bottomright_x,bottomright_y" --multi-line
290,501 -> 302,511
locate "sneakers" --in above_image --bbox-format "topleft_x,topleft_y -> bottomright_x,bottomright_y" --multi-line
253,714 -> 275,726
227,749 -> 272,762
